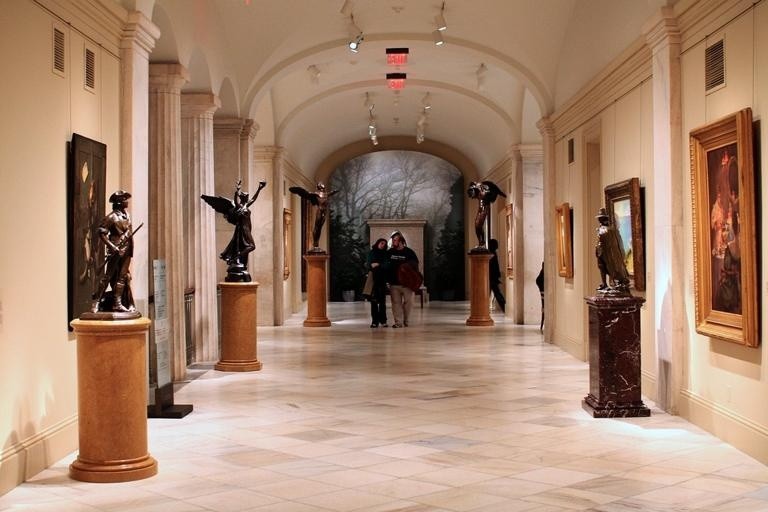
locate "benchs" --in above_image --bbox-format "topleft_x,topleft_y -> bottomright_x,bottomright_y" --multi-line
387,287 -> 425,309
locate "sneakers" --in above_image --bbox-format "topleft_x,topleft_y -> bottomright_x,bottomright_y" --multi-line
370,324 -> 378,327
392,323 -> 402,328
382,324 -> 388,327
404,323 -> 408,327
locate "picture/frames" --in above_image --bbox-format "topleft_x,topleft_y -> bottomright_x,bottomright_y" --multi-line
504,203 -> 514,280
603,177 -> 646,292
687,105 -> 761,348
554,203 -> 573,278
283,207 -> 291,279
67,132 -> 108,334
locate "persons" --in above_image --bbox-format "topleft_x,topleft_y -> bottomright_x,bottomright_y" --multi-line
385,232 -> 419,328
91,190 -> 135,314
220,176 -> 266,266
306,181 -> 335,251
488,239 -> 506,313
594,207 -> 629,290
368,237 -> 391,327
474,181 -> 488,249
536,260 -> 545,332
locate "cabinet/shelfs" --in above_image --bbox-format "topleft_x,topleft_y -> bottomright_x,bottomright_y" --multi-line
364,218 -> 426,287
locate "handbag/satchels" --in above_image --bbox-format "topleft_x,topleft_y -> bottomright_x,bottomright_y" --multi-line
396,263 -> 423,289
363,271 -> 373,295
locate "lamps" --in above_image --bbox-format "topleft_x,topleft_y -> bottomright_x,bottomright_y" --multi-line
307,65 -> 321,80
365,93 -> 380,147
476,63 -> 488,77
384,72 -> 405,90
415,89 -> 433,146
385,47 -> 408,66
432,0 -> 448,48
341,0 -> 366,55
